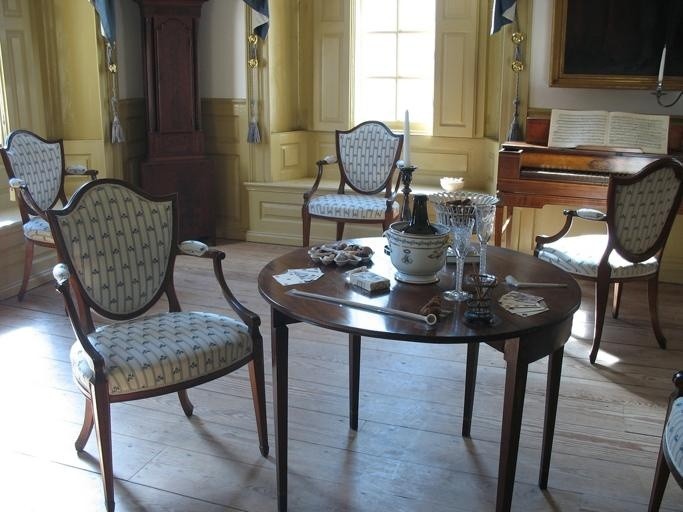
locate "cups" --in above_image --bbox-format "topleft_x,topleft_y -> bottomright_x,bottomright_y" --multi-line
382,221 -> 450,284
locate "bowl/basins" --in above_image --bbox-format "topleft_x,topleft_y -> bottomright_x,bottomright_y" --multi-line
439,178 -> 464,192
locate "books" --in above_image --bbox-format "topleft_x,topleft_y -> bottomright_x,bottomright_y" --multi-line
545,108 -> 671,155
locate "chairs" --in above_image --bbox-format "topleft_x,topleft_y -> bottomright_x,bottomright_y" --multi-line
43,178 -> 271,512
299,120 -> 404,248
0,127 -> 99,317
530,155 -> 681,366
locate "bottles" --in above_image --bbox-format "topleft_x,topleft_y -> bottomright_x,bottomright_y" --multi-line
402,194 -> 436,234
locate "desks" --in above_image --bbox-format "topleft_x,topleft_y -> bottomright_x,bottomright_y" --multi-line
255,236 -> 583,512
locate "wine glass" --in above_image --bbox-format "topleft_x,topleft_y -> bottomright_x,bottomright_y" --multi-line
468,205 -> 497,285
430,192 -> 501,254
442,218 -> 473,301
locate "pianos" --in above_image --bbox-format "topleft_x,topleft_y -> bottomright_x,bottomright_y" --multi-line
495,117 -> 682,248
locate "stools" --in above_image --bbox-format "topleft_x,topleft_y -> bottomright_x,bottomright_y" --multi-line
645,370 -> 682,512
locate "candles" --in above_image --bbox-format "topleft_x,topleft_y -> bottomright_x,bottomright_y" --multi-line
657,43 -> 665,82
403,108 -> 411,168
